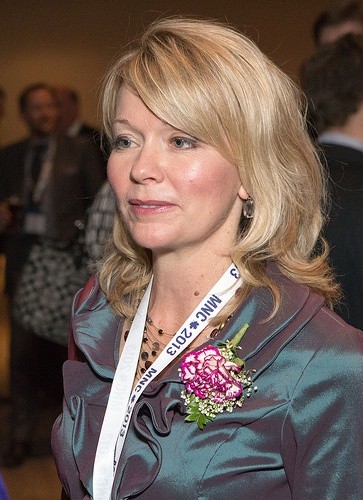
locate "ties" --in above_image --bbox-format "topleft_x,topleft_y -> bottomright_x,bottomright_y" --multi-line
32,144 -> 43,182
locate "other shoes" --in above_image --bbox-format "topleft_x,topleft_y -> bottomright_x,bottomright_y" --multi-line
5,439 -> 46,466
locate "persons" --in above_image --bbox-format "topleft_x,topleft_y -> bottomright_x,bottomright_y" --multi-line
50,17 -> 363,500
0,82 -> 112,469
295,1 -> 363,331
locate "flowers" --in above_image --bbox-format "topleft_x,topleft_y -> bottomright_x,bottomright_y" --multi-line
177,322 -> 258,430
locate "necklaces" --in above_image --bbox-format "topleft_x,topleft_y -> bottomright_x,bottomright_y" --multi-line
124,299 -> 233,381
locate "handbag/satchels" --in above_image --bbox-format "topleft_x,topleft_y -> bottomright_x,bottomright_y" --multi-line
11,244 -> 90,347
